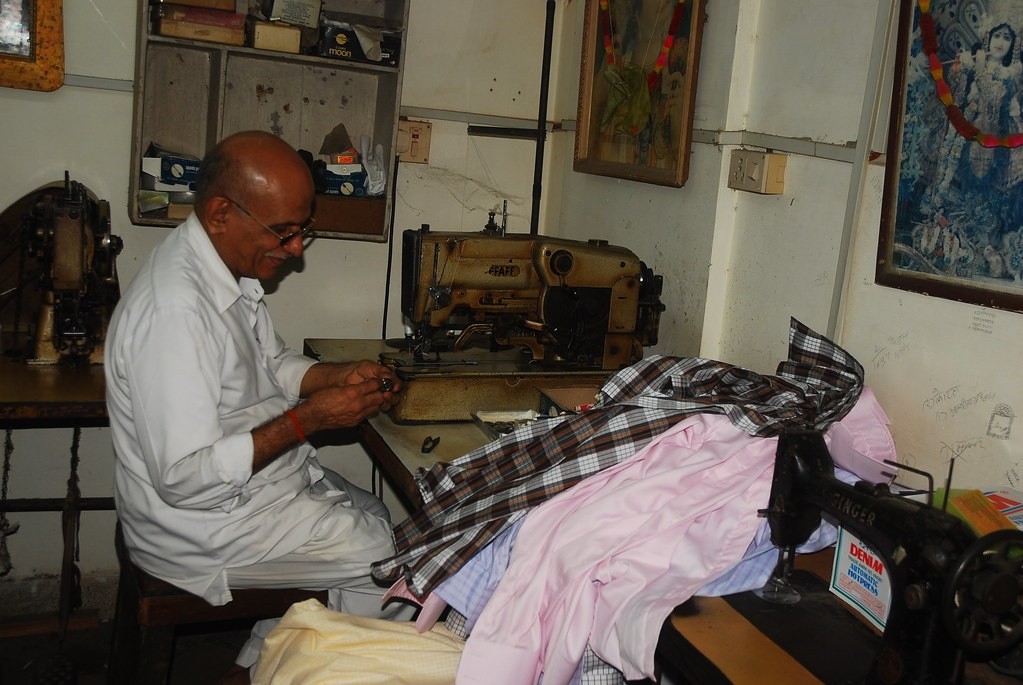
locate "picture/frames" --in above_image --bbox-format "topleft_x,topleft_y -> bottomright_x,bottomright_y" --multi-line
574,0 -> 710,186
870,1 -> 1023,315
0,0 -> 67,92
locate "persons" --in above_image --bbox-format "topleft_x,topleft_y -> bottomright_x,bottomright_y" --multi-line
102,131 -> 398,621
927,25 -> 1023,252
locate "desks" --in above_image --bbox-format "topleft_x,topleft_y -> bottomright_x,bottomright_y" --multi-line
0,332 -> 113,641
302,332 -> 488,517
652,543 -> 1023,685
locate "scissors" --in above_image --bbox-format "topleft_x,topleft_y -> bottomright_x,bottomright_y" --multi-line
421,436 -> 440,452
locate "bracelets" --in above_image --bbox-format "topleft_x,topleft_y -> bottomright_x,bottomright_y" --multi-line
286,406 -> 305,444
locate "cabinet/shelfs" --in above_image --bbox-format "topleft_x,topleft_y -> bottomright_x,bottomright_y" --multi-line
130,0 -> 409,244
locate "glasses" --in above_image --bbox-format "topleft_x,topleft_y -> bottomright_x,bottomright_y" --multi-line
229,199 -> 317,248
